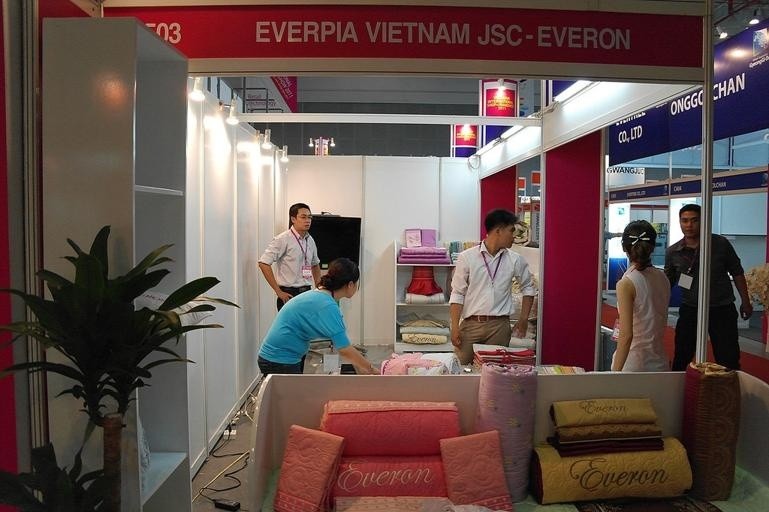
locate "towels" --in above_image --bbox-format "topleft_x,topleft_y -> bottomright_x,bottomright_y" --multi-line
397,228 -> 451,264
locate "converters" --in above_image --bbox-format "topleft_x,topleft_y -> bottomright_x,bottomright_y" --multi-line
214,498 -> 241,510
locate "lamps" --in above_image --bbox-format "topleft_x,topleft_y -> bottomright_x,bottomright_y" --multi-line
186,73 -> 291,164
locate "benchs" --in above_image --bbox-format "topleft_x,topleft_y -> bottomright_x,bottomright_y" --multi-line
243,370 -> 768,512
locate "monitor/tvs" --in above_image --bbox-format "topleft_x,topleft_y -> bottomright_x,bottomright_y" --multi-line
289,215 -> 361,270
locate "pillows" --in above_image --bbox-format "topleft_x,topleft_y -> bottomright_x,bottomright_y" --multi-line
320,400 -> 460,458
529,437 -> 694,506
329,458 -> 452,507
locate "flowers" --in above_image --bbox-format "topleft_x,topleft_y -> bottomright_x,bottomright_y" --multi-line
744,265 -> 769,311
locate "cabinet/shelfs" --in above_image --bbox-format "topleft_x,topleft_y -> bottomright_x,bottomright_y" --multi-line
40,14 -> 192,511
394,240 -> 457,354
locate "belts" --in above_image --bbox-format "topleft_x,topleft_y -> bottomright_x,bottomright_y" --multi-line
470,315 -> 501,323
298,287 -> 310,293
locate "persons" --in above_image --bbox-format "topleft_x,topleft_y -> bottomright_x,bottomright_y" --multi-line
607,220 -> 672,371
664,204 -> 753,371
449,209 -> 538,364
258,203 -> 321,312
257,257 -> 380,378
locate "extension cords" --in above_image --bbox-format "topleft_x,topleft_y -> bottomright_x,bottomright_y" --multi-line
223,429 -> 237,440
